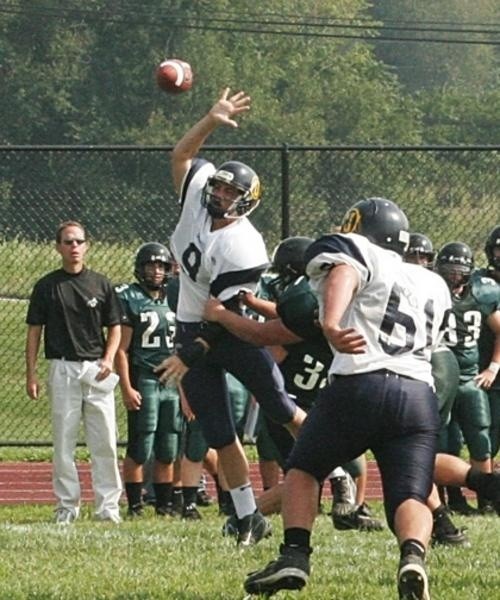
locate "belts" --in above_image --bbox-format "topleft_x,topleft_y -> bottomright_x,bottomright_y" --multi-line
57,355 -> 97,362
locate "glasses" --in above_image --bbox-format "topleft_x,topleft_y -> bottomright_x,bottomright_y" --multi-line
58,238 -> 88,245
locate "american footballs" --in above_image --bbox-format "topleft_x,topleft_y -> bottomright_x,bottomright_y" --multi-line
155,56 -> 195,96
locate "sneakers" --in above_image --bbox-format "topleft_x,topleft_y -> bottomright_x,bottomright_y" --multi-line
333,504 -> 383,532
128,505 -> 145,519
198,491 -> 212,507
95,510 -> 119,523
243,545 -> 314,594
156,505 -> 177,518
236,511 -> 273,545
477,496 -> 495,514
435,525 -> 468,545
55,509 -> 74,523
330,476 -> 352,515
218,500 -> 235,516
397,554 -> 430,600
490,471 -> 500,517
450,501 -> 484,516
180,505 -> 202,520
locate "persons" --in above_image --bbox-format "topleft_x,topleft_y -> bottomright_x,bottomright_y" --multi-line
429,348 -> 500,544
116,243 -> 182,516
471,226 -> 499,281
175,255 -> 250,520
426,243 -> 499,515
170,86 -> 357,543
255,237 -> 379,531
244,198 -> 457,599
28,220 -> 121,523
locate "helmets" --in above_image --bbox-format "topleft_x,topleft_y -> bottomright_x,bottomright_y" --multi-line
200,161 -> 263,220
268,235 -> 315,299
436,242 -> 473,288
341,197 -> 410,258
133,242 -> 173,290
484,225 -> 500,271
